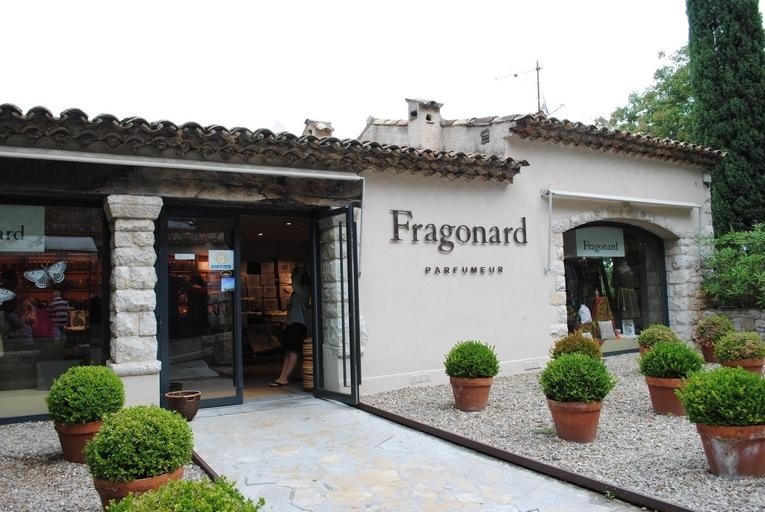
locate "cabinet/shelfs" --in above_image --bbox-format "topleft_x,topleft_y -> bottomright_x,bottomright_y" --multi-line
0,248 -> 233,336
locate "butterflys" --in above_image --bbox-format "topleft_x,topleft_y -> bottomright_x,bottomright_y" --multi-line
24,261 -> 67,289
0,283 -> 16,305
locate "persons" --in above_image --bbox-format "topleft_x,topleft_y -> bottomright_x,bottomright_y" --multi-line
613,262 -> 641,319
186,272 -> 209,332
268,267 -> 310,387
7,301 -> 36,347
48,289 -> 70,342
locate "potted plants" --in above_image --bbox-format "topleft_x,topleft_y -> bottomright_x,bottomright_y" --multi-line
673,363 -> 765,480
47,365 -> 126,464
537,351 -> 620,443
692,315 -> 735,363
443,338 -> 502,412
713,329 -> 765,376
81,404 -> 196,512
638,324 -> 682,358
635,340 -> 705,416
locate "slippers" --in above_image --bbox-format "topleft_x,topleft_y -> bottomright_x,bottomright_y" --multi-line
268,380 -> 289,387
